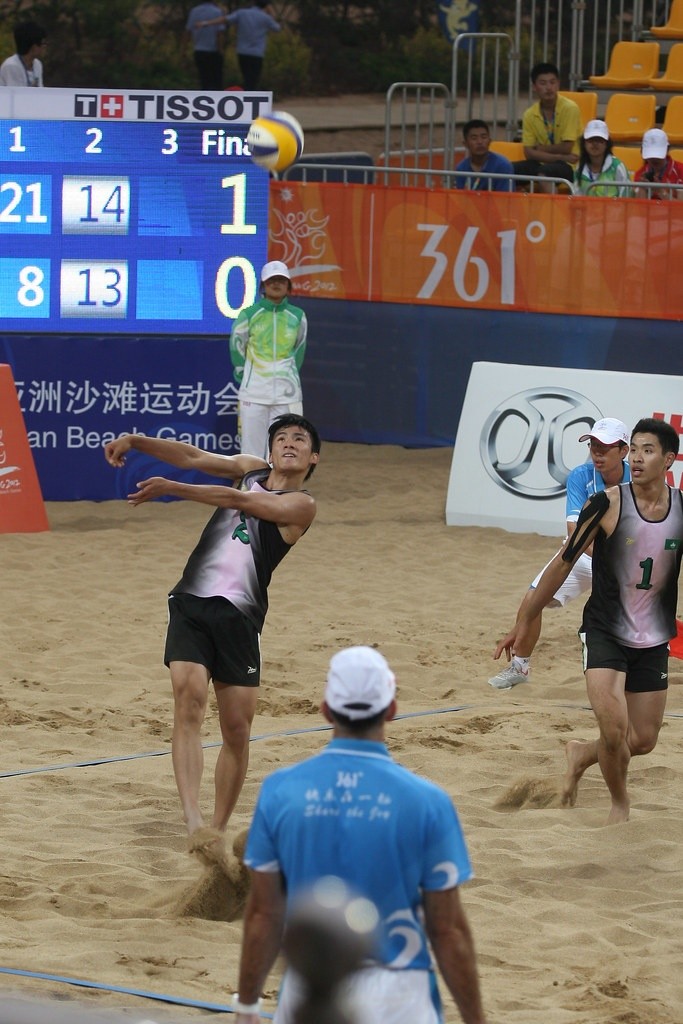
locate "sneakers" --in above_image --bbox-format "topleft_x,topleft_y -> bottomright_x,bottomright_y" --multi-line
488,658 -> 529,690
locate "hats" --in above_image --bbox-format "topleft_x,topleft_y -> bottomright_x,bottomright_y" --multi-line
261,260 -> 290,282
579,418 -> 630,446
584,120 -> 609,143
324,645 -> 397,721
641,128 -> 670,160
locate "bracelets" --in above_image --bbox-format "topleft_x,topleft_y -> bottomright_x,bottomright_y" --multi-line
202,21 -> 208,28
233,994 -> 264,1016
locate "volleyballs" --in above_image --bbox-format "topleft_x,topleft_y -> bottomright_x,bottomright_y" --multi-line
246,109 -> 306,171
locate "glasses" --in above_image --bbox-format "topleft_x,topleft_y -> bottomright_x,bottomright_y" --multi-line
266,277 -> 285,286
587,442 -> 620,450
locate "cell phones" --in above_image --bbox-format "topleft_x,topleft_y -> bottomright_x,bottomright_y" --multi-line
644,172 -> 655,182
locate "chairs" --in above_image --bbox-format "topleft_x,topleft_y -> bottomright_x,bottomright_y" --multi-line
486,0 -> 683,184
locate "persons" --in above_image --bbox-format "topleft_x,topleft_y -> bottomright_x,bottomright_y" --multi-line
194,0 -> 281,90
0,22 -> 49,87
572,120 -> 633,197
485,424 -> 633,690
230,261 -> 308,469
511,62 -> 585,196
631,127 -> 683,202
233,646 -> 489,1024
104,412 -> 321,866
453,119 -> 516,192
494,418 -> 683,825
186,0 -> 226,90
